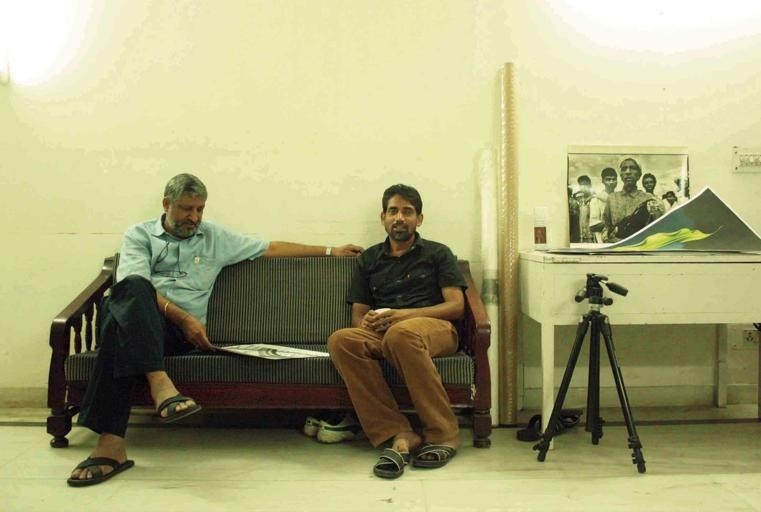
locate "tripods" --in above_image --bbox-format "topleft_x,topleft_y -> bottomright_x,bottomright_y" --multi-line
535,272 -> 649,474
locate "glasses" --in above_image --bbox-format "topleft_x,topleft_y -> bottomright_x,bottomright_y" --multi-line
154,269 -> 188,278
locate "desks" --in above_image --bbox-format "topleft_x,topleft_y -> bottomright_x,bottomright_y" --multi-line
516,248 -> 761,451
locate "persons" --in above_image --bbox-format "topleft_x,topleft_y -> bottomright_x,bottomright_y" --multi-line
567,156 -> 680,244
67,174 -> 366,486
328,184 -> 468,460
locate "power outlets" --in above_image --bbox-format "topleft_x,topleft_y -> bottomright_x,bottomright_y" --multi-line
743,329 -> 759,346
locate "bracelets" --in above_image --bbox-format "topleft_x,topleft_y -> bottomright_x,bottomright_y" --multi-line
163,300 -> 171,320
325,247 -> 332,256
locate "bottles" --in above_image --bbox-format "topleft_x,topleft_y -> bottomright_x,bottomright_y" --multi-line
534,207 -> 552,251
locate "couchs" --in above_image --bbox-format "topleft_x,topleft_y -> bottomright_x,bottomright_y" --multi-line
44,248 -> 497,452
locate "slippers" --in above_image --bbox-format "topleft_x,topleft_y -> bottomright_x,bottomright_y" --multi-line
413,444 -> 456,469
517,409 -> 584,443
373,447 -> 411,478
156,393 -> 201,423
67,454 -> 134,487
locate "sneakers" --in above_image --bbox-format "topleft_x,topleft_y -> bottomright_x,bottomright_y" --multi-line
304,412 -> 362,443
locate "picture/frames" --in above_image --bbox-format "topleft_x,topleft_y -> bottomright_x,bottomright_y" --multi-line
560,142 -> 693,251
